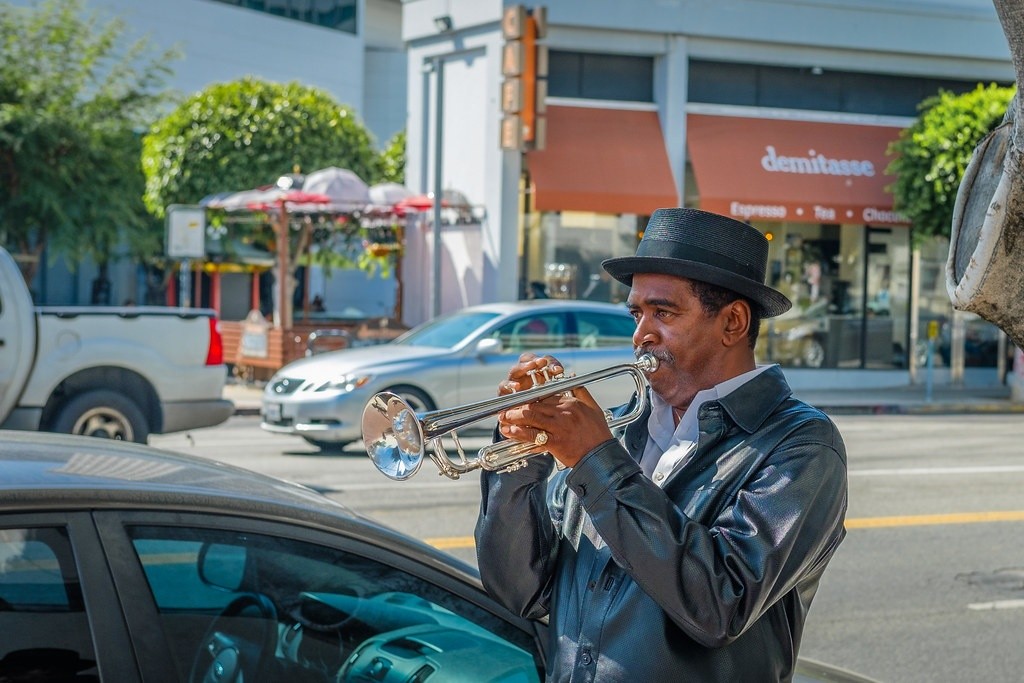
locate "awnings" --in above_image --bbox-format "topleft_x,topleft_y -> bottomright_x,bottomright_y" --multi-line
525,100 -> 939,226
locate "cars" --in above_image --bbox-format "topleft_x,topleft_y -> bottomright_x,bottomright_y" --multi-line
761,296 -> 946,370
0,430 -> 872,683
258,300 -> 645,452
934,313 -> 1016,369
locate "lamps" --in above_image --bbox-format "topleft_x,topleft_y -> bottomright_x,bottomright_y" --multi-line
433,15 -> 451,32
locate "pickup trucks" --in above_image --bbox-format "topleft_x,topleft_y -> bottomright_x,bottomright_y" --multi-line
0,246 -> 239,447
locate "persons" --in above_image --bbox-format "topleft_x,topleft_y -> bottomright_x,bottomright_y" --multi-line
474,207 -> 848,683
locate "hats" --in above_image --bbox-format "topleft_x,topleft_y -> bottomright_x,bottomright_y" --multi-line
601,208 -> 792,319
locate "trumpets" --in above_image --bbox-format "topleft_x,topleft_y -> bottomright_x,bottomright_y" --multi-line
362,351 -> 661,482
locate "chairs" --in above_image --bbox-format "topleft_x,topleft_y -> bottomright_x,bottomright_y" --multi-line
516,319 -> 545,349
